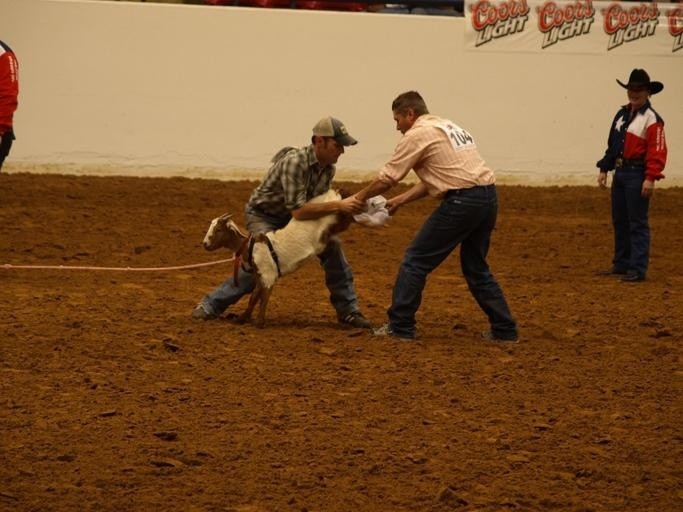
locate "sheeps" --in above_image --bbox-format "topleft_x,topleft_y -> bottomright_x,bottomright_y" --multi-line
201,173 -> 368,330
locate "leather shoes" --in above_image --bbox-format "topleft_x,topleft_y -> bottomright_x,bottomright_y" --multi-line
620,270 -> 641,281
598,265 -> 628,275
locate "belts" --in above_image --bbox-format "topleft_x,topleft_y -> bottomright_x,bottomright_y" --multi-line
615,158 -> 643,168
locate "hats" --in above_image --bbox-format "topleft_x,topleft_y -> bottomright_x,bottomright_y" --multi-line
616,68 -> 663,95
312,116 -> 358,147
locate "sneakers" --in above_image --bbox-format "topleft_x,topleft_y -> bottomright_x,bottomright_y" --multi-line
373,324 -> 413,343
476,330 -> 520,343
338,310 -> 371,328
192,304 -> 211,320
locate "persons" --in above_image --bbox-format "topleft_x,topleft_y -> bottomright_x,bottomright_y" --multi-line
595,67 -> 667,283
340,89 -> 521,346
0,40 -> 18,171
190,115 -> 373,331
